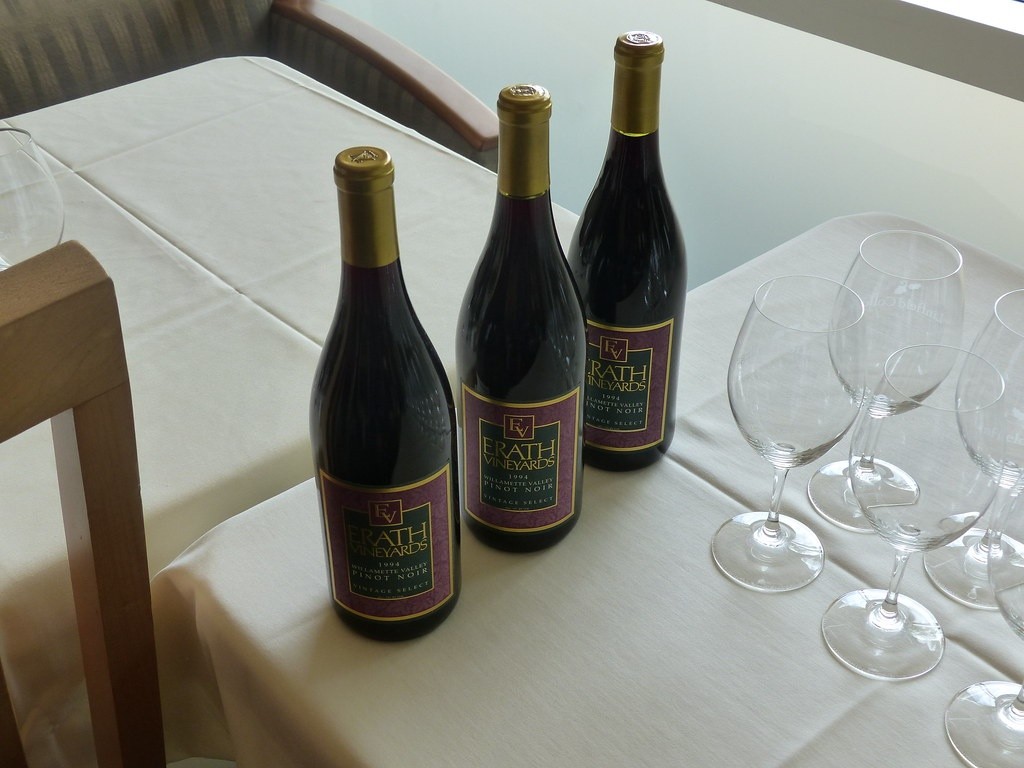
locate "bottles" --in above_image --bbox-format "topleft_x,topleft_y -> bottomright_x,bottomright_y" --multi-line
455,84 -> 589,558
568,32 -> 691,475
307,141 -> 462,645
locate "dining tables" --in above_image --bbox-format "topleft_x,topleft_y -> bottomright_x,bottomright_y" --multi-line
176,206 -> 1024,768
1,56 -> 581,768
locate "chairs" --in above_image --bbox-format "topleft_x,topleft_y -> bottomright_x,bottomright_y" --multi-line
0,0 -> 499,175
0,242 -> 171,768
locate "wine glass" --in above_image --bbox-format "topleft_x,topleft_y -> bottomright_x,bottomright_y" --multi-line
820,342 -> 1009,683
943,485 -> 1024,768
924,287 -> 1024,612
807,230 -> 967,536
709,274 -> 868,594
